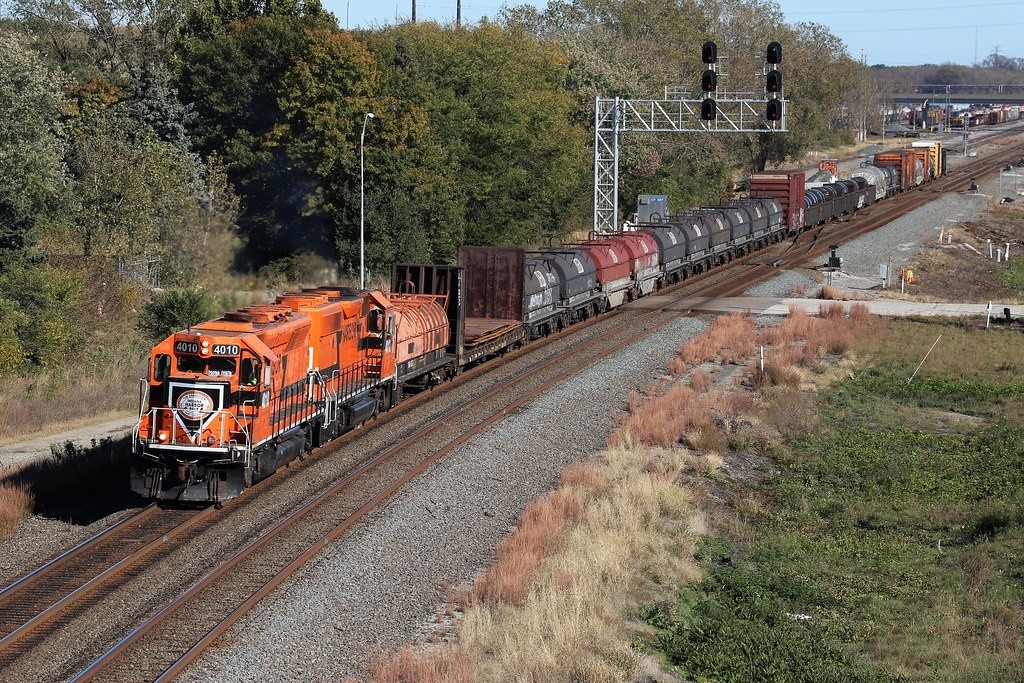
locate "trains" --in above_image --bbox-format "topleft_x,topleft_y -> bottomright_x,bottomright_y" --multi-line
126,139 -> 949,505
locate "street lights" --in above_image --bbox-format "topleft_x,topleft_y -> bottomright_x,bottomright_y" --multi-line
359,111 -> 375,291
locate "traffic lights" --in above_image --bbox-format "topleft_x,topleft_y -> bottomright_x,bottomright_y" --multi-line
765,70 -> 782,92
766,41 -> 783,63
701,98 -> 716,120
701,41 -> 717,64
701,70 -> 716,91
766,98 -> 782,120
